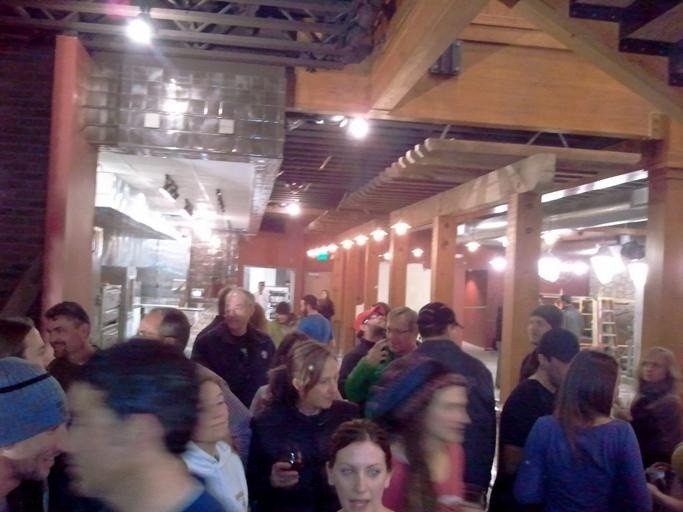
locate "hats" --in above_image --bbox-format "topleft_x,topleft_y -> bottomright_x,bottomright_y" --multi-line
353,305 -> 385,333
417,302 -> 464,332
531,305 -> 563,328
0,358 -> 69,447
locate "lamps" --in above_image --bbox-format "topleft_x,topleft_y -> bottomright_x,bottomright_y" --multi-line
168,191 -> 180,202
125,5 -> 157,43
306,218 -> 424,260
590,239 -> 617,284
465,226 -> 481,252
183,198 -> 194,216
538,249 -> 561,283
159,174 -> 178,197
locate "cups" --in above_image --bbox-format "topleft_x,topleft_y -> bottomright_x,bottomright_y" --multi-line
279,442 -> 304,476
646,467 -> 665,482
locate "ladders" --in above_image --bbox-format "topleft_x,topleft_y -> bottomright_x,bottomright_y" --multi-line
598,296 -> 616,368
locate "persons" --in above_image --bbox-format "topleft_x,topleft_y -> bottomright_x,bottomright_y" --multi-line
488,293 -> 683,512
0,281 -> 495,511
